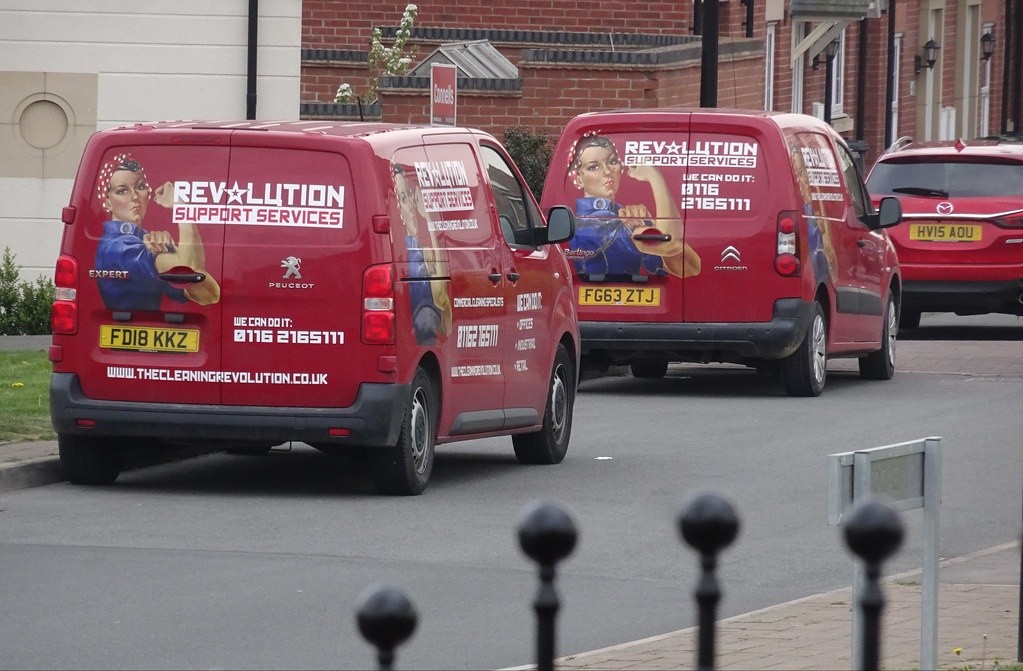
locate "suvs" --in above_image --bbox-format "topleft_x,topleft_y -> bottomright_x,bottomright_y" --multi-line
861,133 -> 1023,329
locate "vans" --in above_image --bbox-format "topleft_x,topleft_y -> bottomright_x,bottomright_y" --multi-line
535,106 -> 904,397
45,117 -> 583,498
